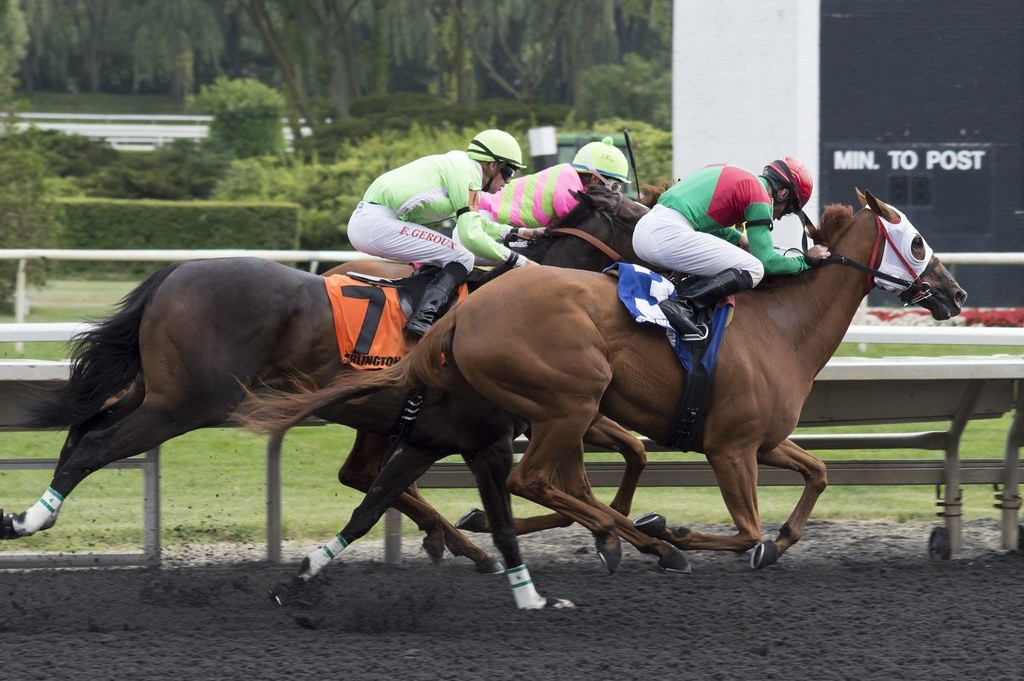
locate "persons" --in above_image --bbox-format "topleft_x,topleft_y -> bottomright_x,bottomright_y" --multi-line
347,129 -> 547,336
632,157 -> 833,348
478,136 -> 631,246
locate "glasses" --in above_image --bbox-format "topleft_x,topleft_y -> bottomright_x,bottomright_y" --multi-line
502,166 -> 515,181
783,199 -> 794,214
595,174 -> 621,193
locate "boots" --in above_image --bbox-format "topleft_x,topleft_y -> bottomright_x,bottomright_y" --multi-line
659,268 -> 741,349
406,269 -> 460,339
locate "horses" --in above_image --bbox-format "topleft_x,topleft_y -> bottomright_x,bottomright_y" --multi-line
0,175 -> 966,619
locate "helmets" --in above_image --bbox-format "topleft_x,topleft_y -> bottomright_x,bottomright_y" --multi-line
763,156 -> 813,209
467,129 -> 527,169
571,136 -> 631,184
